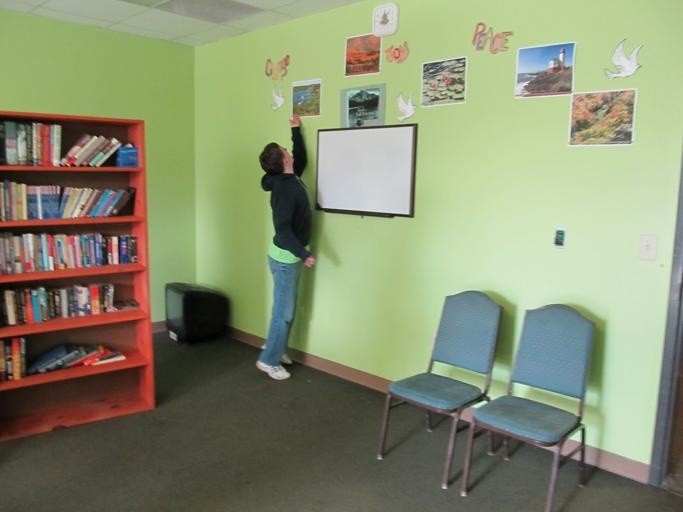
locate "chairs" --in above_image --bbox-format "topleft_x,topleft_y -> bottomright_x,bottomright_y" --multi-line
459,303 -> 596,512
377,290 -> 504,490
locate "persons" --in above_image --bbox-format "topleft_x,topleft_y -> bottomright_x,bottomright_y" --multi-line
256,114 -> 317,380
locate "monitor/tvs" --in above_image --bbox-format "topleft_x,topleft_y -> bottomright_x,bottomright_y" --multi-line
163,281 -> 230,348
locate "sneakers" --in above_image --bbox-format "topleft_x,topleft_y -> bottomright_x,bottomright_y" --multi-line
260,344 -> 293,365
255,360 -> 292,381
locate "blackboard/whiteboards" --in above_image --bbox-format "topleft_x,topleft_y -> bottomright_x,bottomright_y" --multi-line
315,123 -> 418,218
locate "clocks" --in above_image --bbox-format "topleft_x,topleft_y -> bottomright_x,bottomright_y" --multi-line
372,3 -> 399,37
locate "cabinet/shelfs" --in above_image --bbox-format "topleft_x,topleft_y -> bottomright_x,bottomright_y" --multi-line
0,110 -> 156,441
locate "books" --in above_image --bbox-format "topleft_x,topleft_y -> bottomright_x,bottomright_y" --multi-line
0,282 -> 139,323
0,121 -> 122,168
0,179 -> 136,221
0,230 -> 138,274
0,337 -> 125,383
